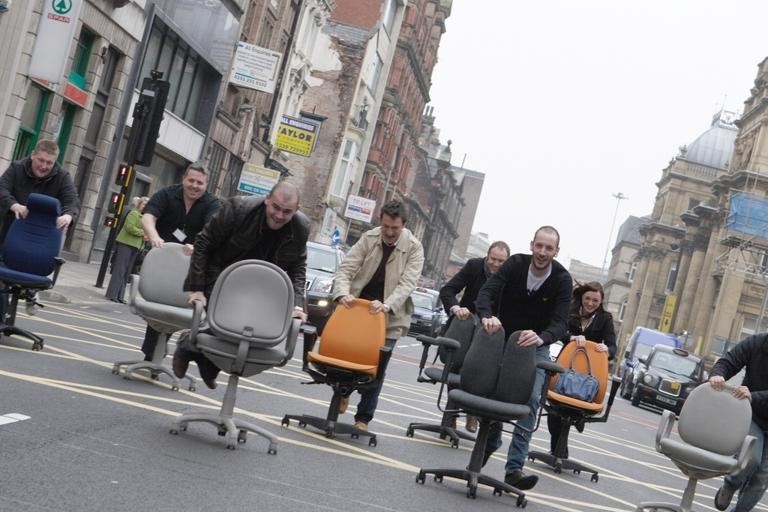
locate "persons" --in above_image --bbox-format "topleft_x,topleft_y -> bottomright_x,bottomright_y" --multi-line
106,193 -> 148,305
137,164 -> 220,363
439,240 -> 510,434
707,331 -> 768,512
330,198 -> 425,431
475,225 -> 571,491
548,281 -> 618,463
174,178 -> 311,392
0,140 -> 82,315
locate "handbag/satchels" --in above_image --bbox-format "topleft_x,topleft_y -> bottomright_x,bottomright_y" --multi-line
554,368 -> 599,403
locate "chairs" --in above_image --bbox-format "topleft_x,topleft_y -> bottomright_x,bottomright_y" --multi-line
635,382 -> 758,512
0,192 -> 66,351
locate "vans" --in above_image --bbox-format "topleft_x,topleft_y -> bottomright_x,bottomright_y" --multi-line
618,326 -> 680,396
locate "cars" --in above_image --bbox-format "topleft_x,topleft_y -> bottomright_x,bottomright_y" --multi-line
407,287 -> 440,339
629,343 -> 703,420
546,339 -> 570,367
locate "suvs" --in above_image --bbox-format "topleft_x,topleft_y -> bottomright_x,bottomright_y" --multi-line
300,239 -> 345,335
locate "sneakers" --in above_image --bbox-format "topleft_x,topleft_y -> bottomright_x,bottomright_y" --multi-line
353,421 -> 367,434
715,486 -> 735,511
120,299 -> 127,303
111,299 -> 118,303
25,292 -> 38,316
339,397 -> 348,413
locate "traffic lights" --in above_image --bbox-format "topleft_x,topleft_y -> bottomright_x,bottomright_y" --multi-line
103,163 -> 132,225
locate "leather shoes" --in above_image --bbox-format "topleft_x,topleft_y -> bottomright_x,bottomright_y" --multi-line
465,414 -> 477,434
172,345 -> 188,378
199,365 -> 217,388
481,444 -> 496,467
505,472 -> 538,490
